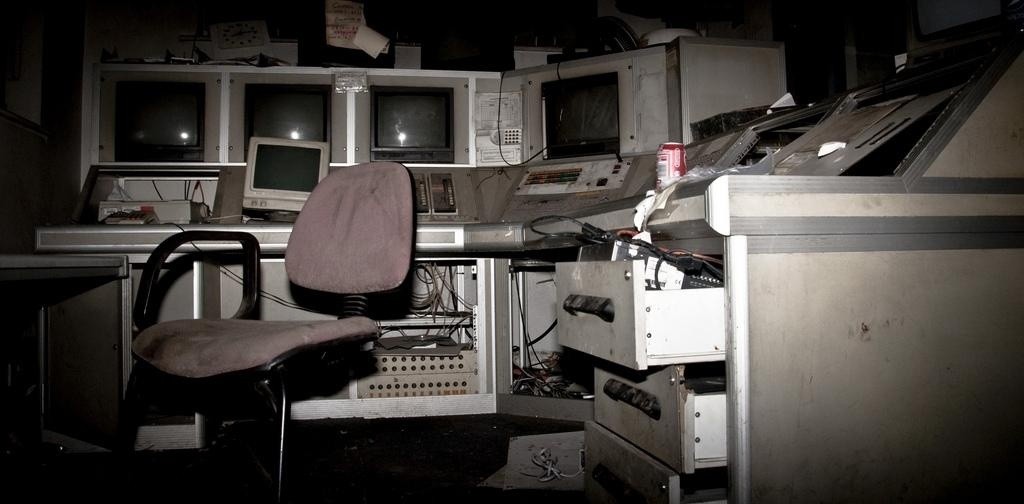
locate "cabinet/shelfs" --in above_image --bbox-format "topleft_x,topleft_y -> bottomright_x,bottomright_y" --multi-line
554,173 -> 1024,503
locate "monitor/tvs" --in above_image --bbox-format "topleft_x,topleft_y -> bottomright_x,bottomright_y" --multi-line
547,77 -> 619,159
242,136 -> 331,223
249,91 -> 325,141
370,85 -> 455,162
116,80 -> 204,160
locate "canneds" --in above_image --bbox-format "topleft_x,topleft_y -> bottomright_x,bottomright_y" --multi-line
656,141 -> 687,192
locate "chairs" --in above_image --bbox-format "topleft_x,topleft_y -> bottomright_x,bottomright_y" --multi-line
105,161 -> 415,504
